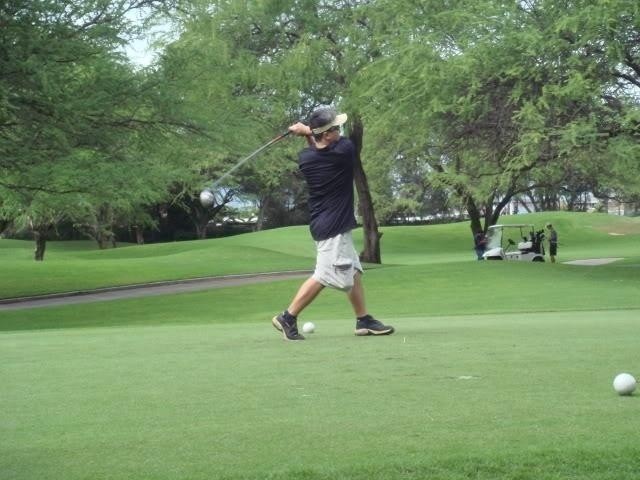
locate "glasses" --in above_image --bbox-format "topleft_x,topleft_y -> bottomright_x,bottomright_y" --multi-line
328,125 -> 340,132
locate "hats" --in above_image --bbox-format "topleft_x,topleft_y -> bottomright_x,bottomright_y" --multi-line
309,108 -> 348,134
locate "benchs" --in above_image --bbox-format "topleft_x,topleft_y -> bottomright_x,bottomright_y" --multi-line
511,241 -> 532,255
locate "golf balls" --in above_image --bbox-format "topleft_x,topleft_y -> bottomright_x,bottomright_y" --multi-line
302,322 -> 315,333
613,372 -> 637,397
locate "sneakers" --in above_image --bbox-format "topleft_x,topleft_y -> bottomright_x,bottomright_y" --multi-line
355,317 -> 393,335
271,310 -> 306,341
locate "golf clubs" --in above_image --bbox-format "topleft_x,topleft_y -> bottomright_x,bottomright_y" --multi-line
200,116 -> 308,210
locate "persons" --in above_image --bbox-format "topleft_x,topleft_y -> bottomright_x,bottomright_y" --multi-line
473,230 -> 488,260
546,223 -> 557,262
271,107 -> 394,340
